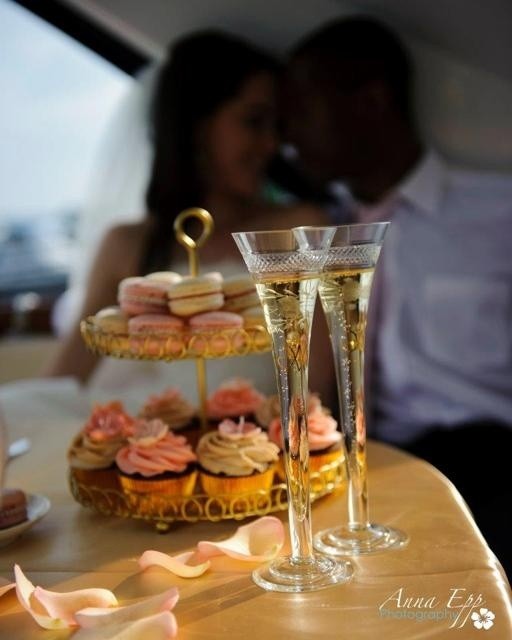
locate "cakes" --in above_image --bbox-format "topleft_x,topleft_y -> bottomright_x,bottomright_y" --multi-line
94,271 -> 264,354
68,385 -> 342,515
0,488 -> 27,527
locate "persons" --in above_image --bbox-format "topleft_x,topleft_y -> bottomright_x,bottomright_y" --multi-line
0,12 -> 512,583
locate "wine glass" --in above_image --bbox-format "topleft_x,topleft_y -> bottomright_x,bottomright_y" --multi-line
230,221 -> 409,593
